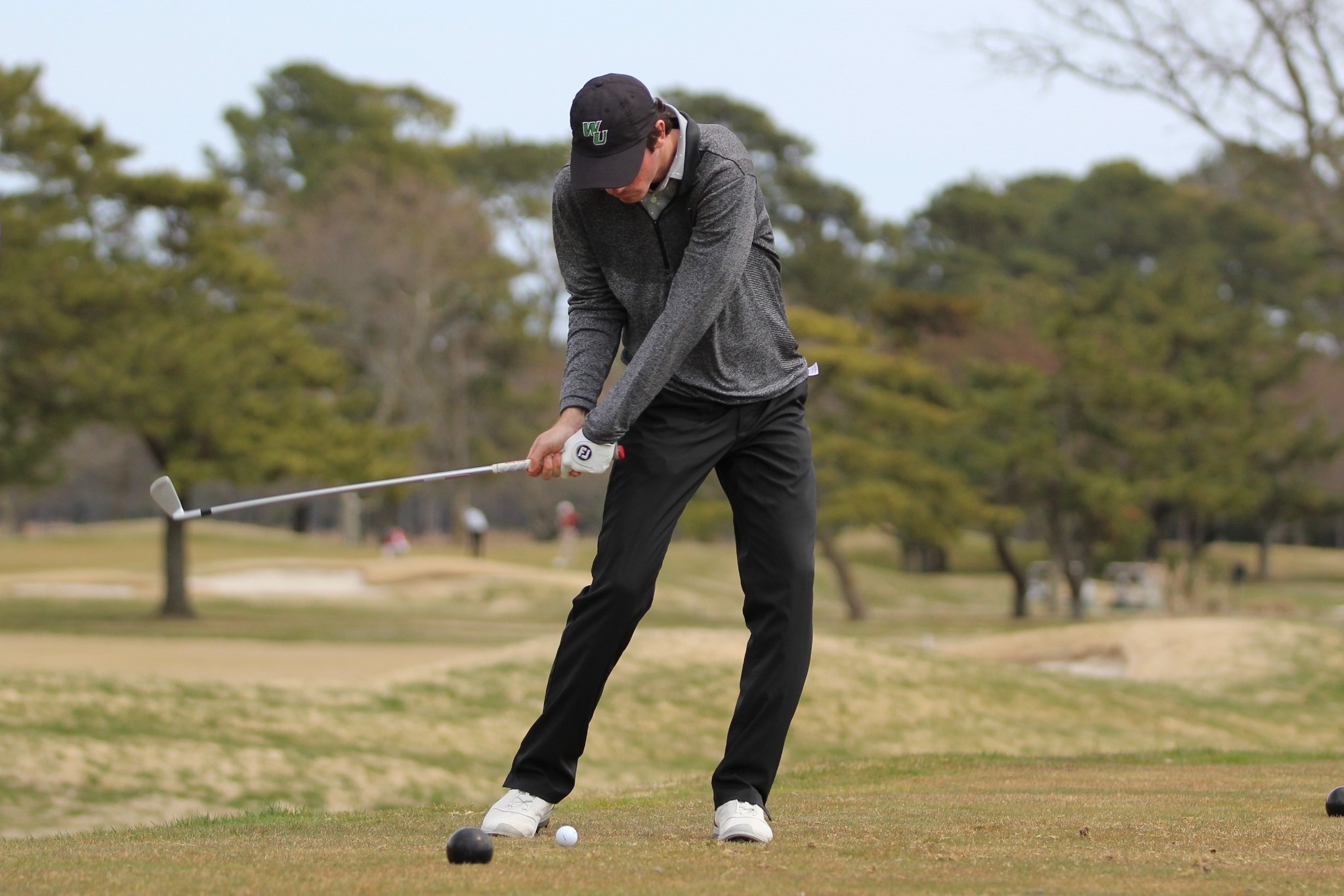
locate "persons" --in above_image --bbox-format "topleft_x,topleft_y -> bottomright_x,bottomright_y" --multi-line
479,75 -> 818,841
383,499 -> 579,556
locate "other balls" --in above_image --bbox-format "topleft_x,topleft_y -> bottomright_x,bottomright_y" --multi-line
1325,785 -> 1344,817
446,826 -> 493,864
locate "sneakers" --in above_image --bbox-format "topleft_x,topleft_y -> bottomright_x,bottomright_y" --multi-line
714,799 -> 772,843
480,789 -> 555,837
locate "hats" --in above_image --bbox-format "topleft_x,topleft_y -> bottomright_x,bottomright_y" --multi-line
570,73 -> 657,190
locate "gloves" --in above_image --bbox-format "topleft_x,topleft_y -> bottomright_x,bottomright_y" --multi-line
560,427 -> 616,479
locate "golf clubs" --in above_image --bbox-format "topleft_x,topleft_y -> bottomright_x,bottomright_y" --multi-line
148,440 -> 625,523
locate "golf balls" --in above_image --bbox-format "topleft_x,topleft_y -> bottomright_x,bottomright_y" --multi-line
555,824 -> 578,847
